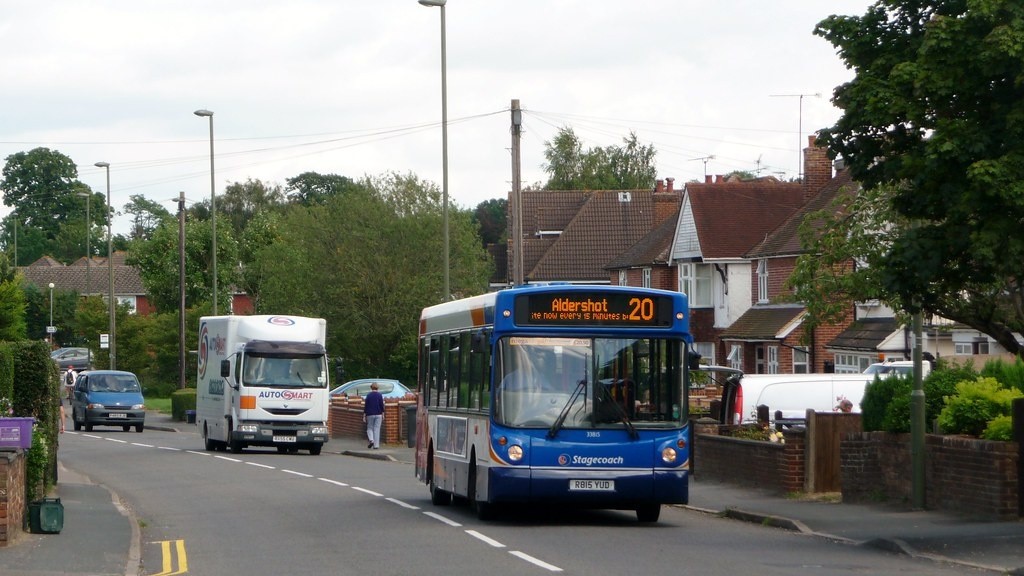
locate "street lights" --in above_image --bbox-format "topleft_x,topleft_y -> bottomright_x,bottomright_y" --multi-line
94,162 -> 116,370
417,0 -> 452,302
76,192 -> 91,296
48,283 -> 56,354
194,110 -> 219,317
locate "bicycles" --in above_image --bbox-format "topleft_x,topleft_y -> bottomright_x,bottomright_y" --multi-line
69,387 -> 73,405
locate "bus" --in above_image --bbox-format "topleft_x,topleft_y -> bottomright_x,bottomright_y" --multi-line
415,281 -> 701,526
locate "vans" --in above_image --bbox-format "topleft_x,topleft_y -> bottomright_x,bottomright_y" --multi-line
863,359 -> 930,384
699,362 -> 905,433
72,370 -> 145,432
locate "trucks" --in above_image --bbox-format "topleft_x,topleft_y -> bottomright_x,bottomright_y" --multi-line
196,315 -> 331,456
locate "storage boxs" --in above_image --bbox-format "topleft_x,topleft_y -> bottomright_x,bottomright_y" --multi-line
0,417 -> 36,447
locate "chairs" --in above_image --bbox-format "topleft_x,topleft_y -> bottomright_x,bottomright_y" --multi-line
291,358 -> 321,386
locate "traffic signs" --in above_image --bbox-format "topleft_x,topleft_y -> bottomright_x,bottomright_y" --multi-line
46,326 -> 57,333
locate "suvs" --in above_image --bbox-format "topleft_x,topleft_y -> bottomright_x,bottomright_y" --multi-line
48,347 -> 94,371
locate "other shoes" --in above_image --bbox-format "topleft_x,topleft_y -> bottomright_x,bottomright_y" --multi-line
368,441 -> 374,448
373,447 -> 378,449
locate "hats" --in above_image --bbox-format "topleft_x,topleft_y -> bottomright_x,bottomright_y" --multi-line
68,366 -> 74,369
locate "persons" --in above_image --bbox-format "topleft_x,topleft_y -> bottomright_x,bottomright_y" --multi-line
60,398 -> 65,434
63,365 -> 78,400
364,383 -> 385,449
496,353 -> 559,404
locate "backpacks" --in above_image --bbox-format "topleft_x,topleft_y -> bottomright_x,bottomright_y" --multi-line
66,371 -> 74,384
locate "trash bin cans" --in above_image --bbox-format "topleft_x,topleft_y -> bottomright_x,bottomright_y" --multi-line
29,496 -> 64,534
0,417 -> 36,450
186,410 -> 196,423
406,408 -> 417,448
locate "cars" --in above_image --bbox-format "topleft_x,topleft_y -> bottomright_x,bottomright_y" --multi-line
328,377 -> 414,402
